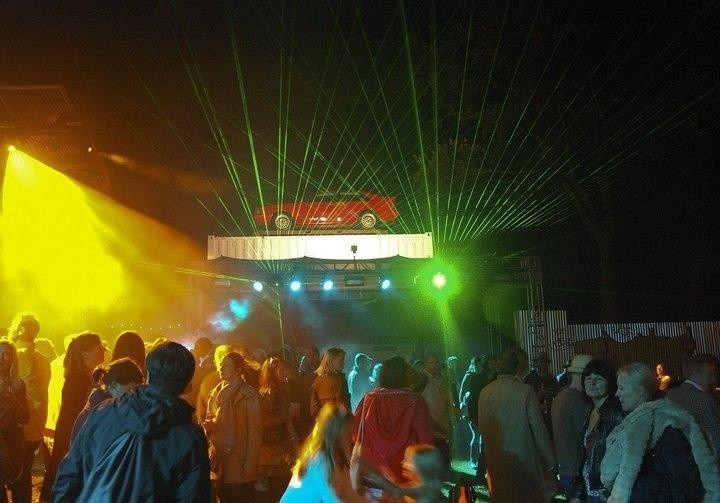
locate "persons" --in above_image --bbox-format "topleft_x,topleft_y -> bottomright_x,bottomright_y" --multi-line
1,313 -> 720,502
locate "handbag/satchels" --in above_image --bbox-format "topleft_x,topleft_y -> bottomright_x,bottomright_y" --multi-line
349,395 -> 367,492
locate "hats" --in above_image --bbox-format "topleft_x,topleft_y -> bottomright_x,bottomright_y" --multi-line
566,354 -> 594,374
531,352 -> 552,363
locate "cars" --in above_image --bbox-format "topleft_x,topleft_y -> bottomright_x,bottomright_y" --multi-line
256,189 -> 399,228
